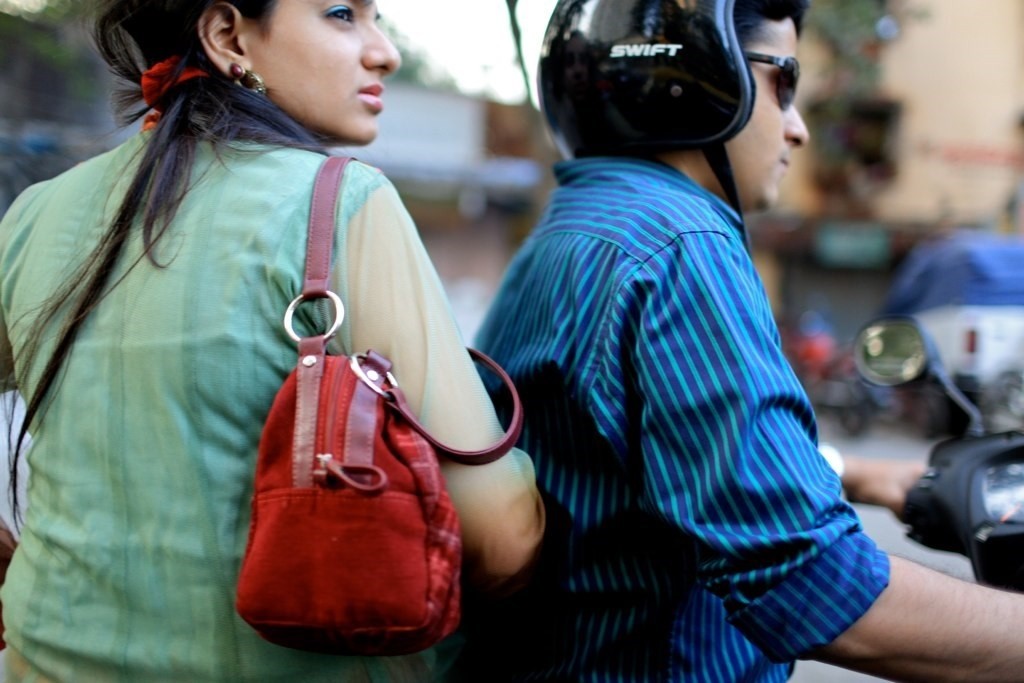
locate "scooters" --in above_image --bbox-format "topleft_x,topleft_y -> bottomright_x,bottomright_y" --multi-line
848,314 -> 1024,597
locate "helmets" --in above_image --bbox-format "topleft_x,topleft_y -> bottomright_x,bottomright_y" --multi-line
533,0 -> 755,164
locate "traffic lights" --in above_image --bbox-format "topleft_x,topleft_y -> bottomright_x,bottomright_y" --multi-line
823,94 -> 903,202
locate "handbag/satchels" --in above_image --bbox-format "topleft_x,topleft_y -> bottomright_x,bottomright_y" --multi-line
234,151 -> 525,660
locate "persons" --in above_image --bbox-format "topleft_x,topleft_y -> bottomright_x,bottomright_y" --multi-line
0,0 -> 546,683
461,0 -> 1023,683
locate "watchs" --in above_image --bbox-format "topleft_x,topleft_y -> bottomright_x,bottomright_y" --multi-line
817,444 -> 844,476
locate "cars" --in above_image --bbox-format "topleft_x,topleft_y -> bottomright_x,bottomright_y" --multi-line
741,214 -> 1004,441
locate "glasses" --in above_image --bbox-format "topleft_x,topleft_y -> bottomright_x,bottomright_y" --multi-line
744,46 -> 802,113
